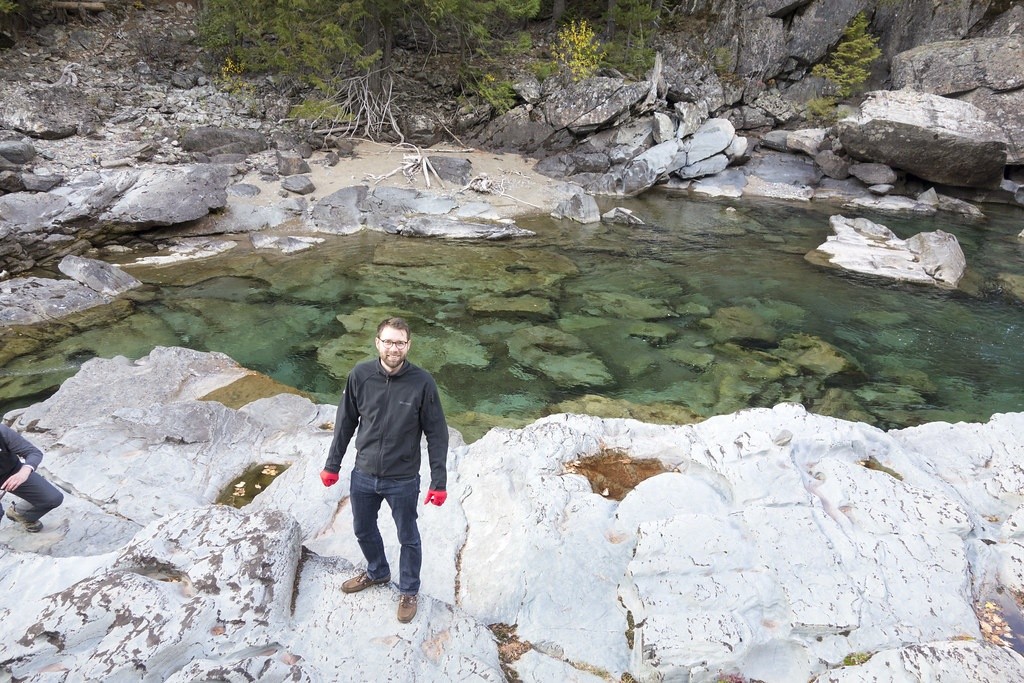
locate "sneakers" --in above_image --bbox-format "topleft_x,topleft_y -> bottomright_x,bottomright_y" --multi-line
397,595 -> 417,623
341,572 -> 391,593
6,501 -> 43,532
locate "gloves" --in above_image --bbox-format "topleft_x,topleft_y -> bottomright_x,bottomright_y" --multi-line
320,470 -> 339,487
424,490 -> 447,506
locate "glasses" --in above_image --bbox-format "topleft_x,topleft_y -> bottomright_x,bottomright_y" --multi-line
378,337 -> 408,349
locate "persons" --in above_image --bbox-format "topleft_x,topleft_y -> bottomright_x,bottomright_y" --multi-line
321,317 -> 449,622
0,423 -> 64,532
